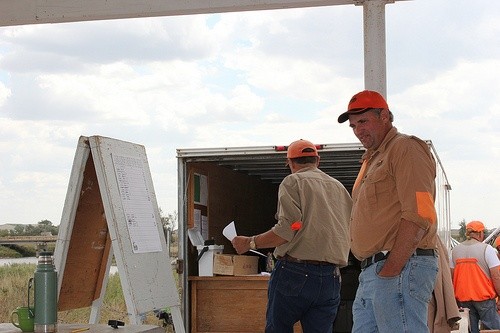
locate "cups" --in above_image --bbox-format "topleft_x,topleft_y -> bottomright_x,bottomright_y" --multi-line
11,306 -> 34,333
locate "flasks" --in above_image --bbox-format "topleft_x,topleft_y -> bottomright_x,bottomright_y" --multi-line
27,252 -> 58,333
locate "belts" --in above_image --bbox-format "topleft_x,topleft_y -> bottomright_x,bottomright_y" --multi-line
286,254 -> 336,267
358,248 -> 434,269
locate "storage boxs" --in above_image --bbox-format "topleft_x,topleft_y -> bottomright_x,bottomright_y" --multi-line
211,253 -> 260,277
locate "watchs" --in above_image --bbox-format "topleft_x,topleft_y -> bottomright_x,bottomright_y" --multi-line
250,236 -> 258,250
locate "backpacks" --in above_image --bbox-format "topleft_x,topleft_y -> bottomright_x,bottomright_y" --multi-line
453,258 -> 498,302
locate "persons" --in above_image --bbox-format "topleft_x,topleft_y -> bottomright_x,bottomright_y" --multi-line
450,221 -> 500,333
231,139 -> 354,333
337,90 -> 439,333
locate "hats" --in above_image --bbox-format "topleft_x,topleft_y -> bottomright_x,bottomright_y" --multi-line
338,90 -> 390,126
496,236 -> 500,246
287,138 -> 319,159
466,221 -> 485,233
